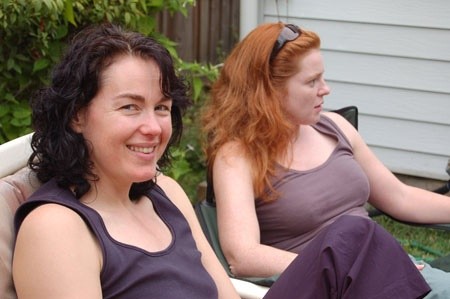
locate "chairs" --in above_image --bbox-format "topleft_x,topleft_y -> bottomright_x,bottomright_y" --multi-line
0,105 -> 359,299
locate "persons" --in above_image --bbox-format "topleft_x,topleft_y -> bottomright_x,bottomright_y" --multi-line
198,23 -> 450,299
12,21 -> 432,299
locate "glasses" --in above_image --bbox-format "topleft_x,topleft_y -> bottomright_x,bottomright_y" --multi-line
268,24 -> 301,65
